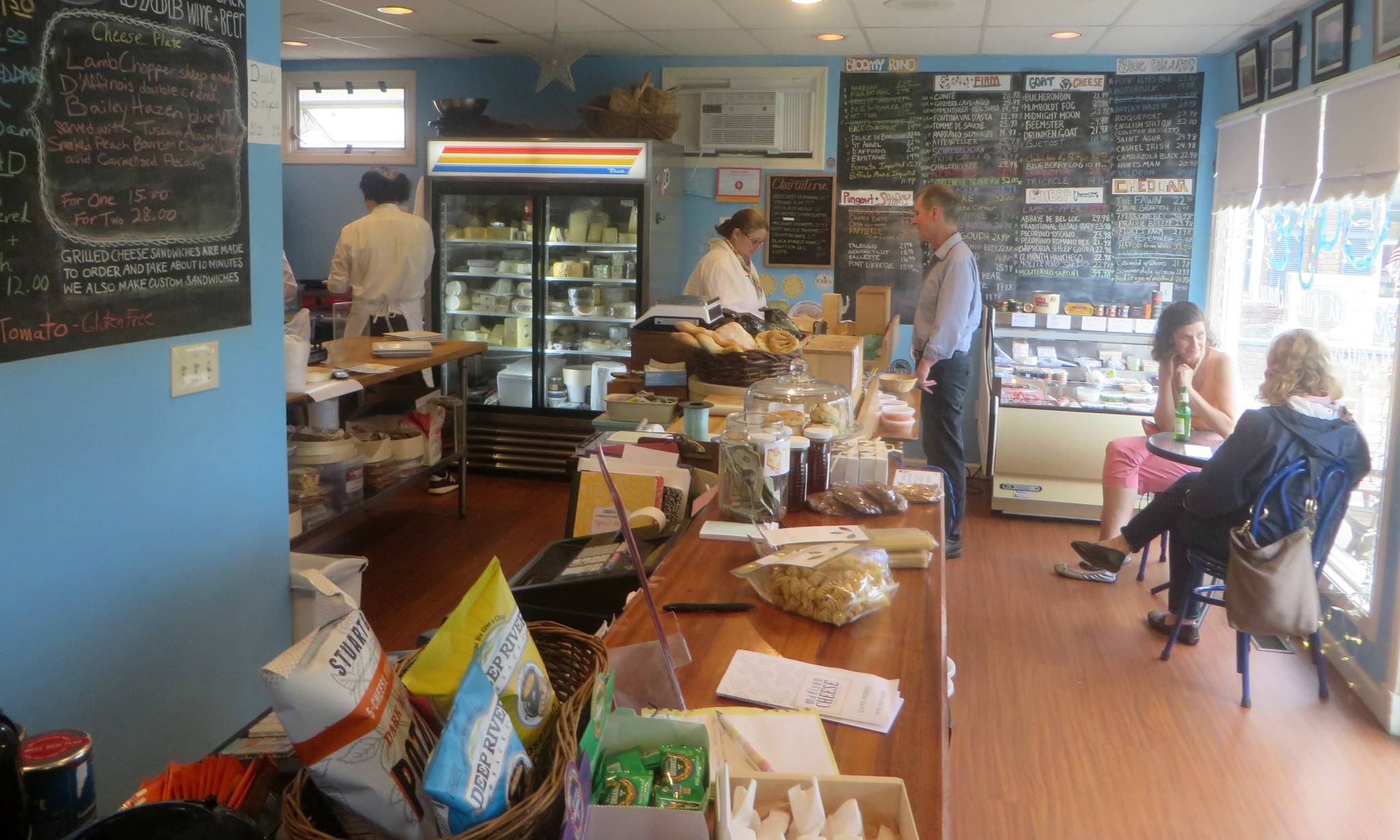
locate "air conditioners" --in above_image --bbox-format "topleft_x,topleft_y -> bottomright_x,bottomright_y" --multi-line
700,90 -> 783,154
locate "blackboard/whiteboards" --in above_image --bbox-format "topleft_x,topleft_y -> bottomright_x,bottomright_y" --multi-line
764,172 -> 837,268
834,71 -> 1205,325
0,0 -> 253,363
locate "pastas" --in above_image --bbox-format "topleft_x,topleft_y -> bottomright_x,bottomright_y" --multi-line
766,553 -> 884,626
855,526 -> 937,569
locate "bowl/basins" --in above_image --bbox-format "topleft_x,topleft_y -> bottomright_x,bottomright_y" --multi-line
432,98 -> 490,117
547,390 -> 567,408
877,373 -> 918,393
864,388 -> 915,434
1076,386 -> 1158,412
315,453 -> 364,507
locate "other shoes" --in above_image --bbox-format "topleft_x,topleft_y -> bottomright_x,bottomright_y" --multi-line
1148,611 -> 1200,646
1079,554 -> 1131,572
1071,540 -> 1125,574
945,539 -> 963,558
1054,562 -> 1117,582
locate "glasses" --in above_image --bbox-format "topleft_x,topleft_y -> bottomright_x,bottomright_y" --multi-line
741,231 -> 765,247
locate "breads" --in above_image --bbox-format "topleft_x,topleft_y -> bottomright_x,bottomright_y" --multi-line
763,402 -> 841,437
672,321 -> 801,353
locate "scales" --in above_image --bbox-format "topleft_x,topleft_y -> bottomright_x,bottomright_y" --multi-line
628,295 -> 724,332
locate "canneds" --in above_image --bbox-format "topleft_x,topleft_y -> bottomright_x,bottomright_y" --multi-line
548,376 -> 563,391
21,728 -> 96,835
1091,302 -> 1130,318
993,299 -> 1033,313
1150,290 -> 1163,319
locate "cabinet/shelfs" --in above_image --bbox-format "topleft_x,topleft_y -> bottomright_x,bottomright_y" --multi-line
286,337 -> 488,553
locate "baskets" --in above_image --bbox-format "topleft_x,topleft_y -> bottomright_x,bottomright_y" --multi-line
688,344 -> 804,387
282,617 -> 613,840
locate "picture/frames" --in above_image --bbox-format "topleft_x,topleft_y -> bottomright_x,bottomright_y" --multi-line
1268,21 -> 1300,99
1236,40 -> 1263,110
1373,0 -> 1400,65
1312,0 -> 1350,84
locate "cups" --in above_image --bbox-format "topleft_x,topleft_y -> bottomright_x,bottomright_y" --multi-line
1040,368 -> 1068,385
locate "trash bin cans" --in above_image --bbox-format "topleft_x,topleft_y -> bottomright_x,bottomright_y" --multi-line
290,551 -> 368,644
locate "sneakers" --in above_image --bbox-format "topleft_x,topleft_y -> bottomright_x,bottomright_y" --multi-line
428,472 -> 460,494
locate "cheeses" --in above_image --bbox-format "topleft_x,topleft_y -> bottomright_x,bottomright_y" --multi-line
445,206 -> 638,351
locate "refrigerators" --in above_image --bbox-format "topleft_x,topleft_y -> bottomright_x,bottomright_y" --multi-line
424,135 -> 684,474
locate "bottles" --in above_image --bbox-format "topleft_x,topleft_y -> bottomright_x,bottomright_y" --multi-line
1173,386 -> 1191,442
744,424 -> 833,517
548,375 -> 565,390
715,412 -> 789,526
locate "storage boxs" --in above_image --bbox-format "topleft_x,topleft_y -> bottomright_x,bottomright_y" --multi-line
564,713 -> 918,840
497,357 -> 567,408
1098,350 -> 1122,360
802,335 -> 864,418
603,393 -> 678,423
856,285 -> 891,334
995,310 -> 1158,334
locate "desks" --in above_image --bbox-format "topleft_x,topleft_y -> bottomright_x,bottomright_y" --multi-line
1146,430 -> 1226,629
602,470 -> 957,840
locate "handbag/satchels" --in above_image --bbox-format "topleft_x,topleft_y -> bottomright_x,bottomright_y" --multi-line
1227,454 -> 1320,636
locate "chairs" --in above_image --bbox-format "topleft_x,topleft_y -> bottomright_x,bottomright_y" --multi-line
1136,419 -> 1348,707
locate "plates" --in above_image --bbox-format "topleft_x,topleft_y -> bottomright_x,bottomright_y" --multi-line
371,341 -> 433,357
383,330 -> 444,344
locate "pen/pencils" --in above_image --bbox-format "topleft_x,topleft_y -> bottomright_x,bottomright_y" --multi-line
663,603 -> 754,612
714,710 -> 774,772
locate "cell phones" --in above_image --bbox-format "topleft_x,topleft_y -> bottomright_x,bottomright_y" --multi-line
332,368 -> 349,379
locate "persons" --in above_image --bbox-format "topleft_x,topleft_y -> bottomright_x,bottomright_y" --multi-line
909,182 -> 982,560
1070,327 -> 1372,647
684,209 -> 769,323
282,249 -> 300,306
325,165 -> 460,493
1055,298 -> 1236,584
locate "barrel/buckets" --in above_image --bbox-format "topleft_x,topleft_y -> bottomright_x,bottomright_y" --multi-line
1032,290 -> 1060,315
562,364 -> 592,404
59,794 -> 266,840
